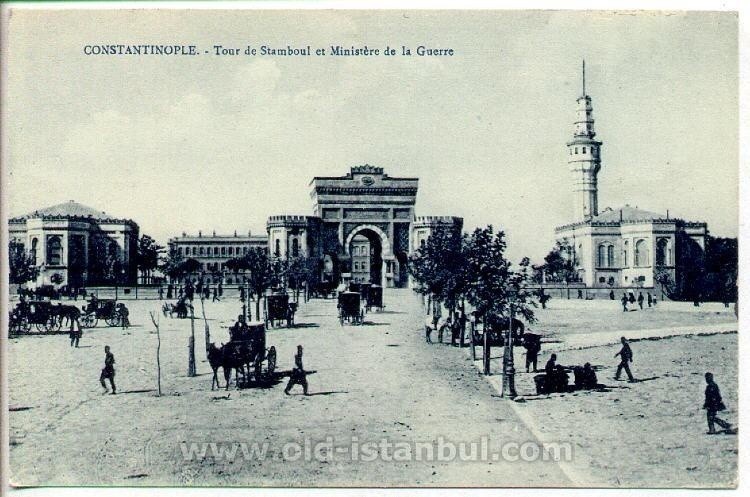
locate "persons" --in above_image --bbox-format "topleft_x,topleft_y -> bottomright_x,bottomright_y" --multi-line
576,287 -> 730,313
613,335 -> 634,380
99,345 -> 117,395
536,352 -> 558,398
584,361 -> 597,389
522,332 -> 541,372
700,371 -> 732,435
9,276 -> 225,346
283,344 -> 316,396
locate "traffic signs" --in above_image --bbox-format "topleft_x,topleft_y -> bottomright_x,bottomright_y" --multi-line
245,278 -> 252,321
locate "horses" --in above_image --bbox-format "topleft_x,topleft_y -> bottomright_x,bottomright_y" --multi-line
487,314 -> 525,341
54,305 -> 81,329
203,342 -> 255,392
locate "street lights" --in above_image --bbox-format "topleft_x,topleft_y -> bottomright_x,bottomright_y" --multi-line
82,272 -> 86,300
302,280 -> 309,303
502,283 -> 518,400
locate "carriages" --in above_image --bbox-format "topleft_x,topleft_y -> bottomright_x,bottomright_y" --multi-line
337,281 -> 386,326
264,292 -> 295,330
207,322 -> 276,391
9,298 -> 120,335
